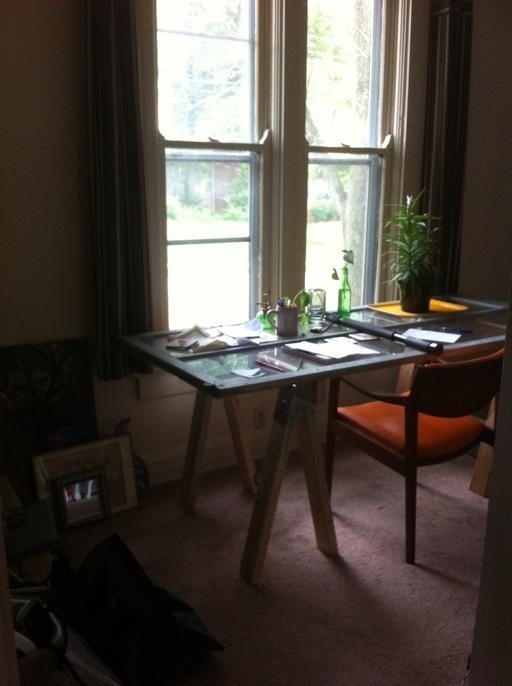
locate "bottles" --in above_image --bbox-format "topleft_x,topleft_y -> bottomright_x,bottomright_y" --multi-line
338,269 -> 351,317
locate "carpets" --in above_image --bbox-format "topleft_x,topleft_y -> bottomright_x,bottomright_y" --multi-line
34,432 -> 511,684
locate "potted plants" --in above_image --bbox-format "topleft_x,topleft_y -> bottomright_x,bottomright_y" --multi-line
332,249 -> 355,315
381,188 -> 439,314
265,290 -> 313,339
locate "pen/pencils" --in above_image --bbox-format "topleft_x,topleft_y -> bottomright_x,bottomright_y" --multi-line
438,326 -> 473,334
245,335 -> 260,339
253,360 -> 285,372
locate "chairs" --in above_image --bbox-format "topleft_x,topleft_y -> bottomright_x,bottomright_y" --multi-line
324,347 -> 506,565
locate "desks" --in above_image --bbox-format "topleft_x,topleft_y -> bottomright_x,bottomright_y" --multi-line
114,296 -> 509,583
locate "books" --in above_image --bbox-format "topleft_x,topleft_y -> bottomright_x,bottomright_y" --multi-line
283,332 -> 381,364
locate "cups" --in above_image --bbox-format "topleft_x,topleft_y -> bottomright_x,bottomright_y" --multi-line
308,289 -> 324,332
266,303 -> 297,338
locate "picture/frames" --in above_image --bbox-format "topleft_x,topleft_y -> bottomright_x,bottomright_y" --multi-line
47,467 -> 113,538
34,435 -> 137,530
